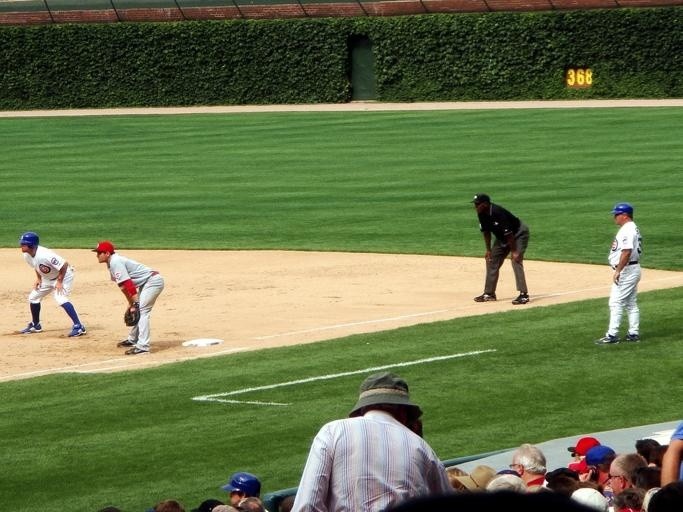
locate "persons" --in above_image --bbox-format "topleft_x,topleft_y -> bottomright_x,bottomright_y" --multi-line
388,423 -> 682,511
292,368 -> 454,512
473,194 -> 529,305
20,232 -> 88,336
92,241 -> 165,355
595,202 -> 642,346
101,472 -> 295,512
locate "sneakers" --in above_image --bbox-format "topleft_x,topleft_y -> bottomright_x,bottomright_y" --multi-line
474,293 -> 496,302
595,335 -> 619,345
21,322 -> 41,333
70,324 -> 86,336
125,348 -> 150,355
512,294 -> 529,304
118,340 -> 137,348
623,334 -> 640,343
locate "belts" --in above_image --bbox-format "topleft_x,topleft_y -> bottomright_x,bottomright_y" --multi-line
613,261 -> 638,270
151,270 -> 159,276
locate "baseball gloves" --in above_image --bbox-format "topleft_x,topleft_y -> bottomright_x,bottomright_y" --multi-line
124,303 -> 139,326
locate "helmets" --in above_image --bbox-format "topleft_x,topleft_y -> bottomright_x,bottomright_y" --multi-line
223,472 -> 260,494
610,204 -> 633,214
19,233 -> 39,245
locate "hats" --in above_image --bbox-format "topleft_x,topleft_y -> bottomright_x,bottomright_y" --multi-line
545,436 -> 617,512
454,467 -> 498,494
92,242 -> 114,254
349,371 -> 422,424
470,194 -> 490,203
